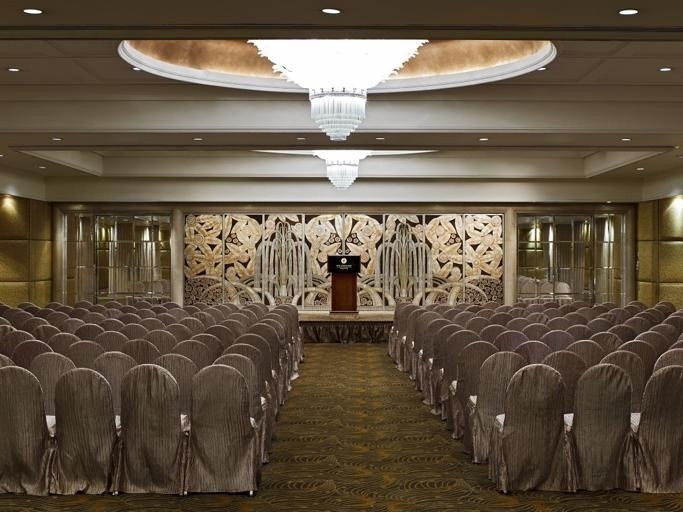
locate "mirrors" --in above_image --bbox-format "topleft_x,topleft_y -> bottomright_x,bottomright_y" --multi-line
61,210 -> 174,304
513,209 -> 627,306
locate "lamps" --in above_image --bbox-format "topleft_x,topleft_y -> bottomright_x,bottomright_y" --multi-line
309,149 -> 373,193
248,36 -> 426,144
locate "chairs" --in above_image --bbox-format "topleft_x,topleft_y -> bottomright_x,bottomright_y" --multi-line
385,298 -> 682,464
48,367 -> 122,498
630,364 -> 683,497
1,365 -> 57,498
563,362 -> 634,493
1,295 -> 302,466
130,277 -> 169,300
514,275 -> 571,302
188,364 -> 259,497
118,362 -> 190,496
487,362 -> 566,493
